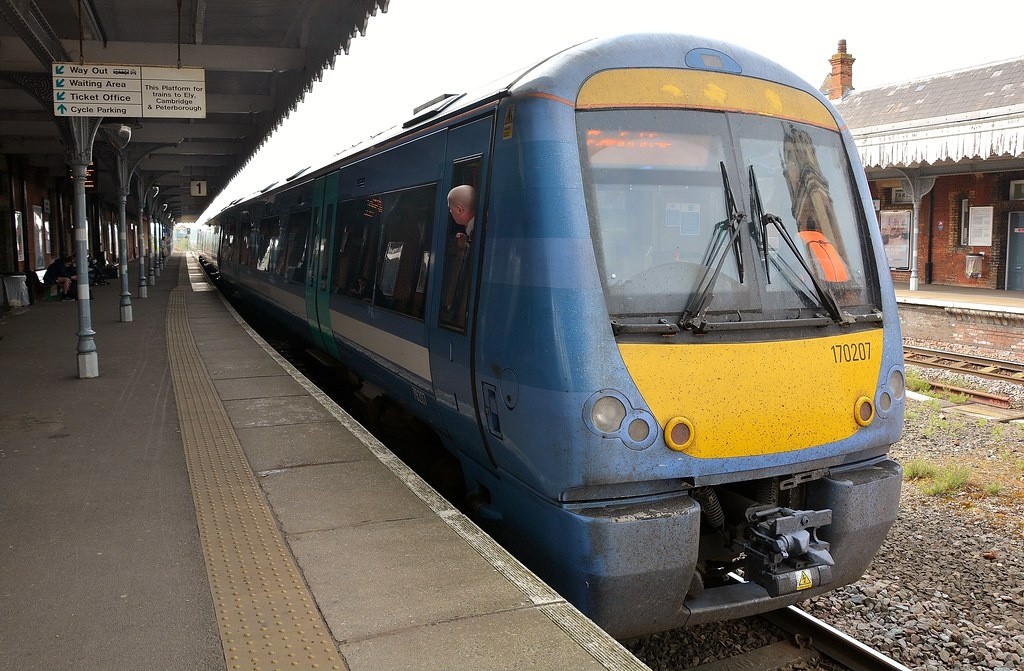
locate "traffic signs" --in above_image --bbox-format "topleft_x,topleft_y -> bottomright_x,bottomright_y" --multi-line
52,62 -> 207,119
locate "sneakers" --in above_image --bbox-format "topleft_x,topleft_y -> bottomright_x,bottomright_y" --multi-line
60,293 -> 75,303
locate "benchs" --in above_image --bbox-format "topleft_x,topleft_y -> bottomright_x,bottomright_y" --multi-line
35,268 -> 63,301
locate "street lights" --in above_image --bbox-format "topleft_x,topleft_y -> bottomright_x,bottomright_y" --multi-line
106,122 -> 177,323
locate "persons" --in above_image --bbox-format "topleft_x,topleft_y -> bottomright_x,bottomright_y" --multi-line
446,184 -> 483,243
43,249 -> 110,303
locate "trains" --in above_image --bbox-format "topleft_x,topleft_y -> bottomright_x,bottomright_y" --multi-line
196,33 -> 906,645
